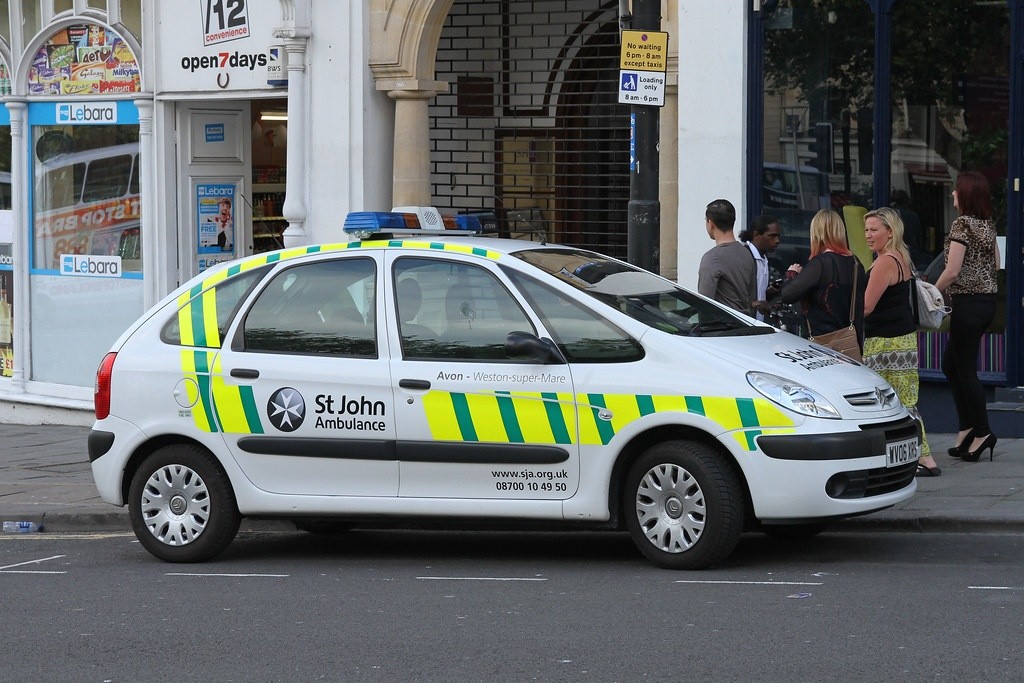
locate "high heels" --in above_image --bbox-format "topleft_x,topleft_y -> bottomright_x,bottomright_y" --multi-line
947,428 -> 977,457
962,433 -> 997,462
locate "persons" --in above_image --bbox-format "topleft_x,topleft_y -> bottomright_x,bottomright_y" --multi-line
698,199 -> 758,330
204,198 -> 234,251
739,219 -> 782,324
861,208 -> 943,476
934,172 -> 1001,463
779,208 -> 867,364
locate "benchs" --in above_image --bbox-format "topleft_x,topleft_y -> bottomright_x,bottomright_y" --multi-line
244,307 -> 367,355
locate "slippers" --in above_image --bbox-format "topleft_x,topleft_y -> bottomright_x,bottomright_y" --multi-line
916,464 -> 941,477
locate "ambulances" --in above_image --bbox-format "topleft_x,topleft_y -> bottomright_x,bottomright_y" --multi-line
87,206 -> 921,570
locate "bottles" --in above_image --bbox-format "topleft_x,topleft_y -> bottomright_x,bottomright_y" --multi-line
0,276 -> 11,343
3,522 -> 42,532
6,68 -> 11,95
0,65 -> 5,95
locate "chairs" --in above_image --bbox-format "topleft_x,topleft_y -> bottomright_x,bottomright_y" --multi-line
440,283 -> 484,340
395,278 -> 440,342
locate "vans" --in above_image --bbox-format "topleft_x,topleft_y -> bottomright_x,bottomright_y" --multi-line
37,141 -> 139,269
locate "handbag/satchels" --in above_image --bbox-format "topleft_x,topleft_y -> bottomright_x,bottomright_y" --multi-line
807,326 -> 862,363
908,259 -> 952,330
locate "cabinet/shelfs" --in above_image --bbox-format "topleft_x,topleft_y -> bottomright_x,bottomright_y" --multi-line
253,184 -> 289,238
